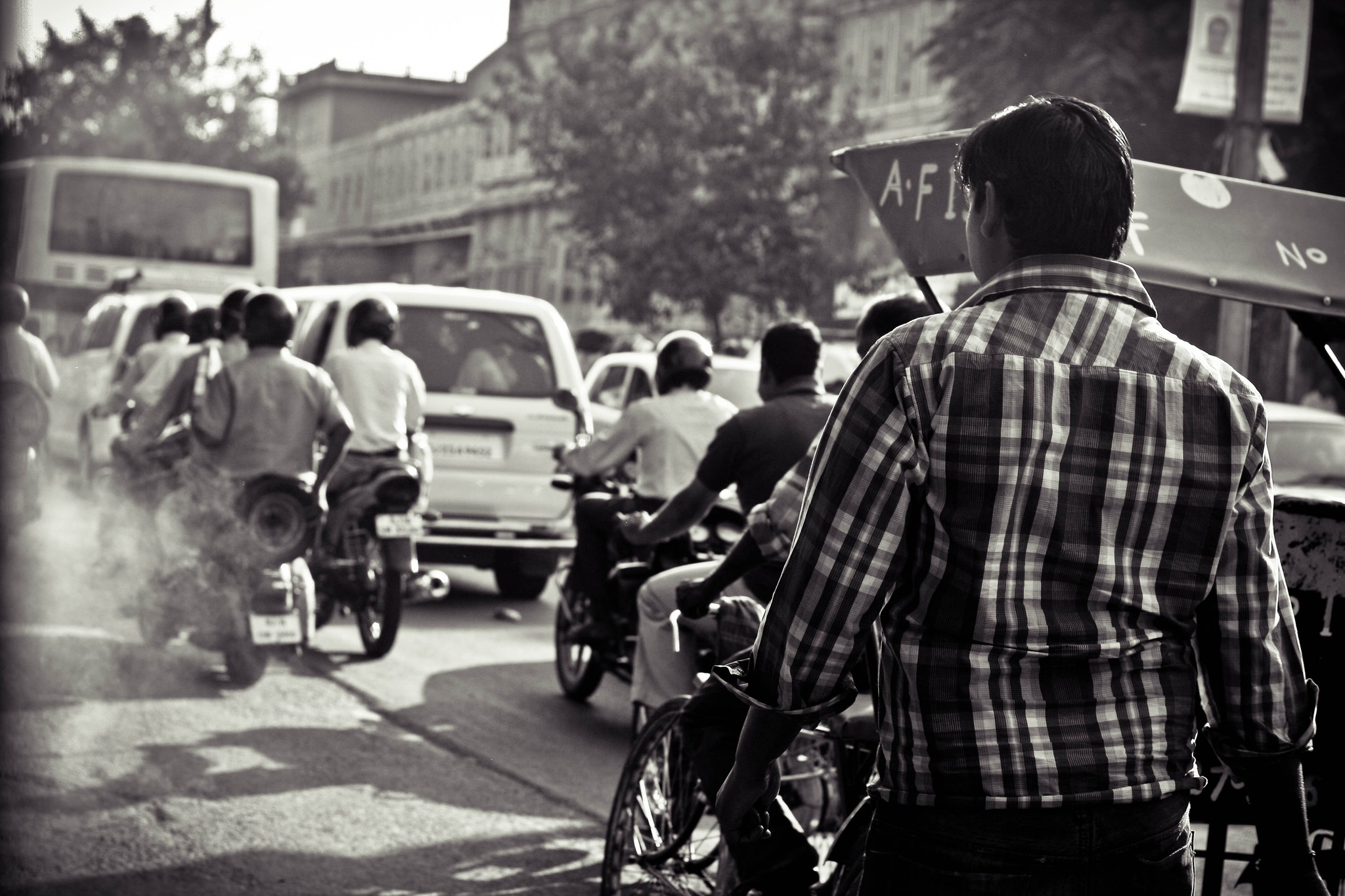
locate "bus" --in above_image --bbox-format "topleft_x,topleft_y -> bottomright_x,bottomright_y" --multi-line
0,155 -> 279,344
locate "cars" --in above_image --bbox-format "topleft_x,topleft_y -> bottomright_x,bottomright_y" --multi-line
586,350 -> 760,436
40,287 -> 220,476
1263,399 -> 1345,501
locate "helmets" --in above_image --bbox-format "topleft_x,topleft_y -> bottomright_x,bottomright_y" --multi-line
654,331 -> 714,394
1,283 -> 30,324
239,294 -> 295,349
219,289 -> 252,335
188,309 -> 219,343
153,299 -> 188,340
346,298 -> 399,348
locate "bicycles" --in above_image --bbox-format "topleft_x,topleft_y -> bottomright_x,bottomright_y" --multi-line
600,596 -> 765,895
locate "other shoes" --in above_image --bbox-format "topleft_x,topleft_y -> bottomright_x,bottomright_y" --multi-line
564,615 -> 619,645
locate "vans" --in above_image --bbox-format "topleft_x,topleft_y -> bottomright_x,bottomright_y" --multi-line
286,279 -> 591,603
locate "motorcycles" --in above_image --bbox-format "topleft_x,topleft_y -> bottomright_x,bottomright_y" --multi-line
112,406 -> 321,686
582,482 -> 877,835
549,387 -> 696,701
310,434 -> 450,657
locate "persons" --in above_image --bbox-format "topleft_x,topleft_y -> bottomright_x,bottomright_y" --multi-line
681,293 -> 937,896
715,96 -> 1327,895
82,292 -> 194,575
0,283 -> 60,522
319,295 -> 433,530
1206,16 -> 1229,52
619,317 -> 839,712
552,337 -> 740,645
109,287 -> 353,561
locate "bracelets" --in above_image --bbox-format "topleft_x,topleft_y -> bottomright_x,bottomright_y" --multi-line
1256,841 -> 1317,862
